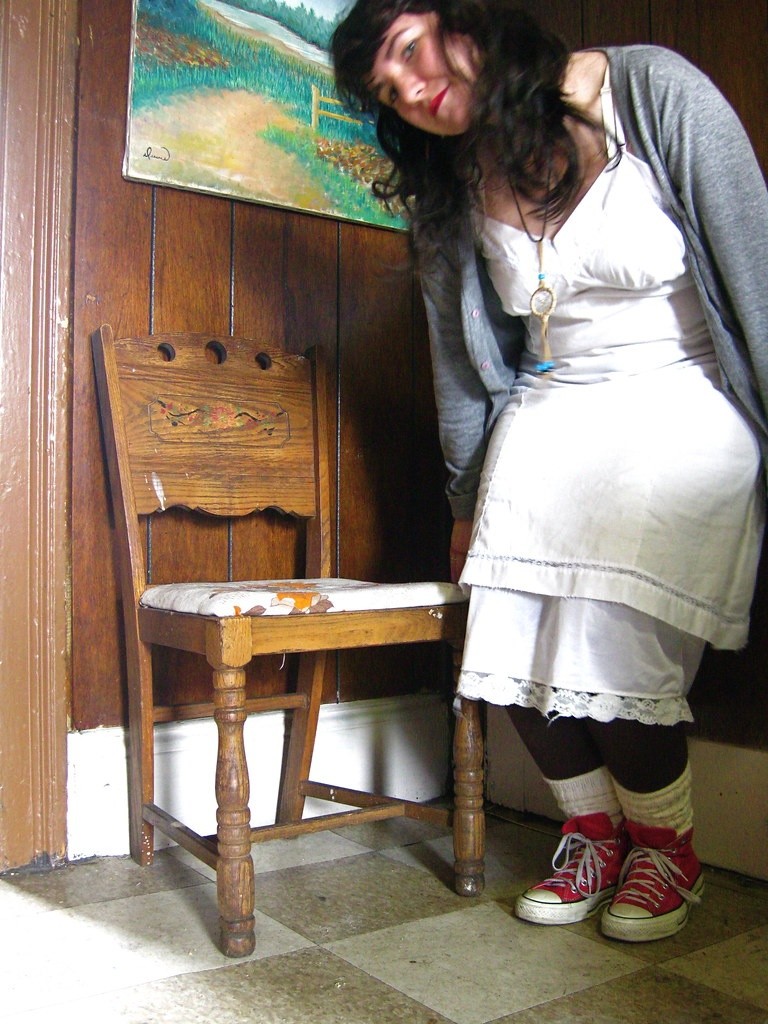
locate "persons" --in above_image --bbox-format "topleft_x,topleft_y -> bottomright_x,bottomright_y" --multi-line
329,0 -> 768,944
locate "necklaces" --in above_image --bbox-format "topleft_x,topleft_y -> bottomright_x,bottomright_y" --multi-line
504,153 -> 556,375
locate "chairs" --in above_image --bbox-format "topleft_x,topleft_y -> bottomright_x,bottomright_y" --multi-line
88,323 -> 488,958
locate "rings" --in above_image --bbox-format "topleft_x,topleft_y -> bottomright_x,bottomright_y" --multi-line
450,558 -> 456,561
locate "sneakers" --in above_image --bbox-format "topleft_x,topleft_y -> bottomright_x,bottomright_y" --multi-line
602,819 -> 705,941
514,814 -> 633,926
